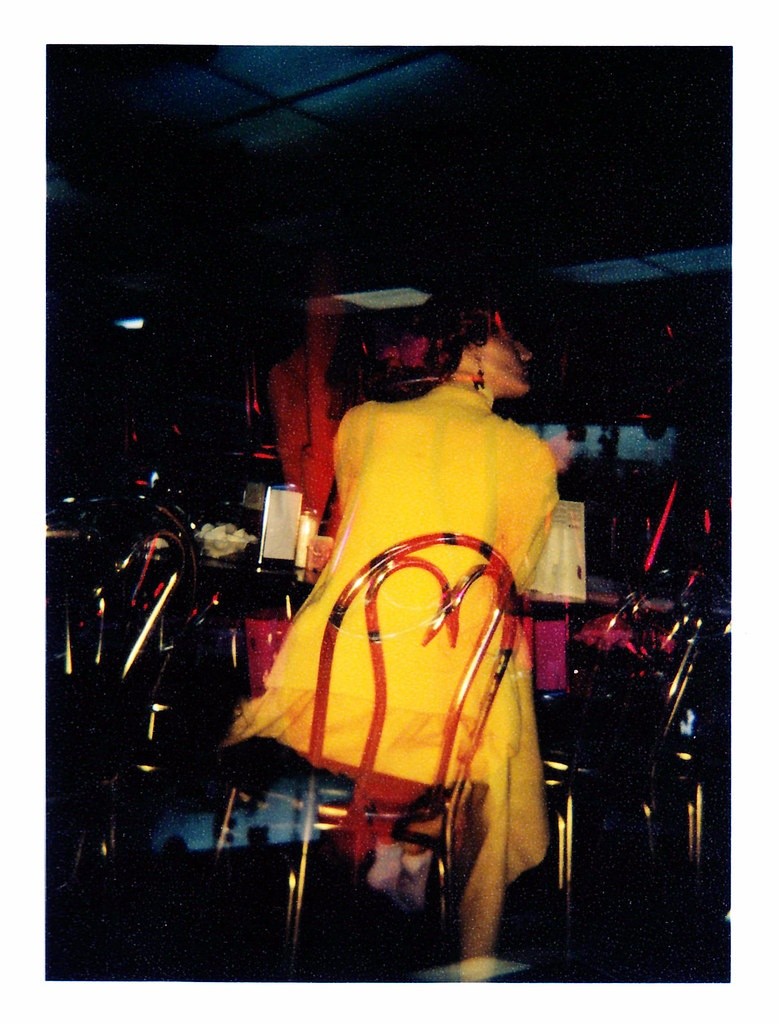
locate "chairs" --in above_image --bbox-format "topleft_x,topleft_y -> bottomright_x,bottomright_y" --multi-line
46,488 -> 730,983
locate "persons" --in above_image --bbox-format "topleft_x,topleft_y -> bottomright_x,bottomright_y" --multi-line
231,289 -> 561,982
